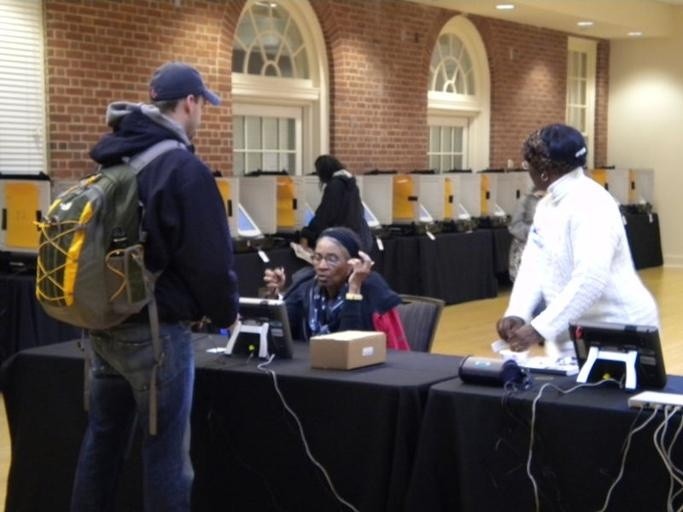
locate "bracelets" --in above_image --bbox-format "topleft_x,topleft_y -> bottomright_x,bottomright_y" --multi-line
345,293 -> 363,300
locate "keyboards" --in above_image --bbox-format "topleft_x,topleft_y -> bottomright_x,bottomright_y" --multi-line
509,354 -> 579,375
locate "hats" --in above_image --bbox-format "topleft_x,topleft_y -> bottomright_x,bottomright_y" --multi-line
523,123 -> 587,171
149,63 -> 220,106
319,227 -> 362,258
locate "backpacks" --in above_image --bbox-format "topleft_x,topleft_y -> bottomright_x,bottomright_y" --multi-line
36,139 -> 186,331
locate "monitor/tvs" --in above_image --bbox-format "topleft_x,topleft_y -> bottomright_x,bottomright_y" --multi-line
421,203 -> 433,223
571,321 -> 668,390
219,296 -> 293,359
362,198 -> 379,230
304,200 -> 317,225
458,201 -> 470,221
238,202 -> 262,238
494,203 -> 505,217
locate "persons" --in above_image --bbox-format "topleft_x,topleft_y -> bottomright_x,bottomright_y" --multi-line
35,60 -> 242,512
300,153 -> 374,254
493,124 -> 659,374
505,182 -> 546,346
262,226 -> 403,343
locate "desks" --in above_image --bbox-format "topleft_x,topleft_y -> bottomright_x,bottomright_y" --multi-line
493,228 -> 515,290
0,331 -> 465,512
622,212 -> 662,270
417,220 -> 493,308
2,223 -> 417,366
405,372 -> 683,512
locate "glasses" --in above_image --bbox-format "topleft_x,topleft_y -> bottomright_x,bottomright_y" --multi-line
522,159 -> 530,168
311,255 -> 349,265
